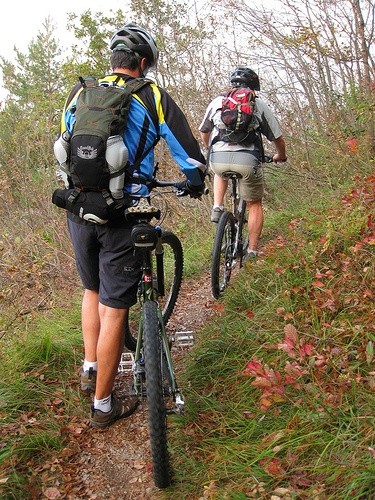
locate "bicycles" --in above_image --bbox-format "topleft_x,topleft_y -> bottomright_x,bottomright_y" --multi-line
200,153 -> 288,300
119,168 -> 212,491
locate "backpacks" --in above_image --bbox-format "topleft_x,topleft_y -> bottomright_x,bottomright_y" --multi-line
212,87 -> 259,144
60,78 -> 154,224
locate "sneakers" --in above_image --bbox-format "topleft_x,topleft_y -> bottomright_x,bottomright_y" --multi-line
80,367 -> 97,396
211,203 -> 224,223
242,252 -> 257,265
91,395 -> 139,430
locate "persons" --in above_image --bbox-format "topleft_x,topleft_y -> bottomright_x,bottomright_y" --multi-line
53,24 -> 208,428
198,67 -> 287,258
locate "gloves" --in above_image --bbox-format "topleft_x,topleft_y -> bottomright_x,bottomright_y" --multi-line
182,180 -> 205,199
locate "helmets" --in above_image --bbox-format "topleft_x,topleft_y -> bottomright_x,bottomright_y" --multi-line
109,25 -> 159,66
231,68 -> 260,91
